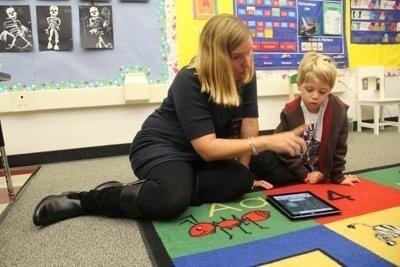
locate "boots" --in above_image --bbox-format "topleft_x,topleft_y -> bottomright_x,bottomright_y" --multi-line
33,177 -> 151,226
95,175 -> 200,206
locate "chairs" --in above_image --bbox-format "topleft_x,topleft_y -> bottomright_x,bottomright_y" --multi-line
355,66 -> 400,135
287,72 -> 300,101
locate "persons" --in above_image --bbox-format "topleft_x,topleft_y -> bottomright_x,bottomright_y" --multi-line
33,12 -> 310,225
249,50 -> 361,188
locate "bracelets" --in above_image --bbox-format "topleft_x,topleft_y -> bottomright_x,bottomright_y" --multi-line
248,135 -> 258,156
